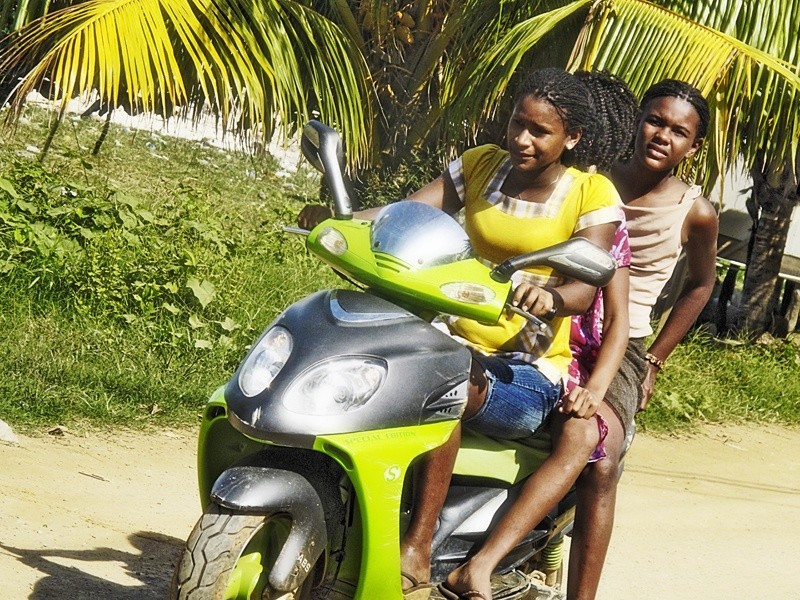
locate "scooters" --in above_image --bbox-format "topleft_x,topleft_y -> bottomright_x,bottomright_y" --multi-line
169,120 -> 636,600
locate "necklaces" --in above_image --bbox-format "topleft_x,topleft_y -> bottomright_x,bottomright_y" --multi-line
509,166 -> 562,200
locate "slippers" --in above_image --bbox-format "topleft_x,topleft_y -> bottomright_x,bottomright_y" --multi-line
438,581 -> 489,600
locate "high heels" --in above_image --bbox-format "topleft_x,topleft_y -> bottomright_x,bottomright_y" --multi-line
400,571 -> 432,600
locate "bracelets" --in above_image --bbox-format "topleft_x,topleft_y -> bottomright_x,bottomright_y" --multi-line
541,287 -> 564,311
640,351 -> 664,371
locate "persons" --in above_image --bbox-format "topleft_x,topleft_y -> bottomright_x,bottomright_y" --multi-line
297,69 -> 624,600
438,71 -> 719,600
